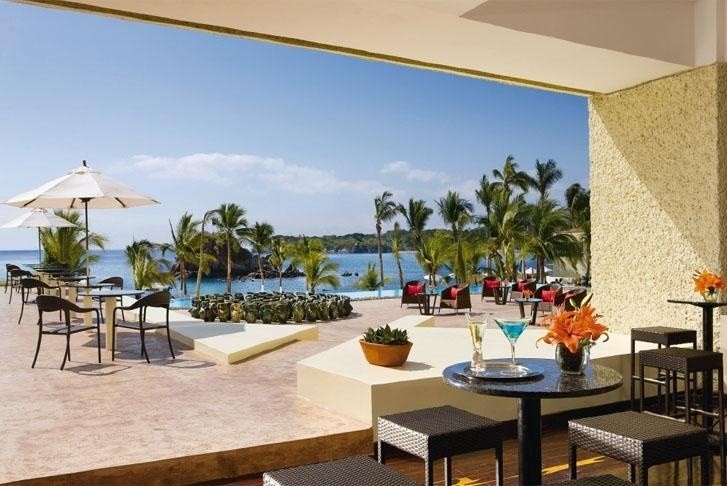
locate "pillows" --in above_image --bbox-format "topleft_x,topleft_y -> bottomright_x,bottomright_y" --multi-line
485,279 -> 499,288
518,283 -> 528,290
409,286 -> 422,296
542,290 -> 557,301
452,287 -> 465,299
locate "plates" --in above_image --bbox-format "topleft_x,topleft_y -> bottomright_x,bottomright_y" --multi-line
455,360 -> 546,381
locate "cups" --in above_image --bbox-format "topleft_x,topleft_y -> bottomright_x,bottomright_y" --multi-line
464,311 -> 489,373
495,318 -> 534,375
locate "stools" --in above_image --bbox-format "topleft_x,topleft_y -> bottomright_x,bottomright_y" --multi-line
639,347 -> 725,481
262,455 -> 416,486
568,409 -> 707,486
630,325 -> 697,416
378,405 -> 503,486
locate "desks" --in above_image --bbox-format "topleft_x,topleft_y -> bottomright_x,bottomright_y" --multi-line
47,275 -> 95,316
76,290 -> 144,351
66,283 -> 114,328
23,260 -> 78,272
668,300 -> 727,428
443,358 -> 623,486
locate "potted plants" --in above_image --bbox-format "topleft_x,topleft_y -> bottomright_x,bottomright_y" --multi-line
359,323 -> 413,367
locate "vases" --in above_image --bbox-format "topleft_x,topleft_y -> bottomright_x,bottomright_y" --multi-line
703,286 -> 718,301
555,342 -> 587,374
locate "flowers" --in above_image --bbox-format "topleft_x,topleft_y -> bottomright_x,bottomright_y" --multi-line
692,270 -> 727,292
537,302 -> 611,351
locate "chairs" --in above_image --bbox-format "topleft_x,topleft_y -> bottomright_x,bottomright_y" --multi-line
112,292 -> 175,363
401,280 -> 426,308
32,295 -> 101,370
4,264 -> 21,293
438,283 -> 471,314
17,279 -> 62,324
481,275 -> 501,301
534,284 -> 562,310
555,288 -> 587,311
98,277 -> 125,322
507,278 -> 536,302
9,269 -> 40,305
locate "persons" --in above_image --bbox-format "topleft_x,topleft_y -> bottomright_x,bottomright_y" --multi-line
550,273 -> 593,286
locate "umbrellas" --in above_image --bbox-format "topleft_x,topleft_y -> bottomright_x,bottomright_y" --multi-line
1,167 -> 163,285
1,204 -> 80,264
424,265 -> 555,284
